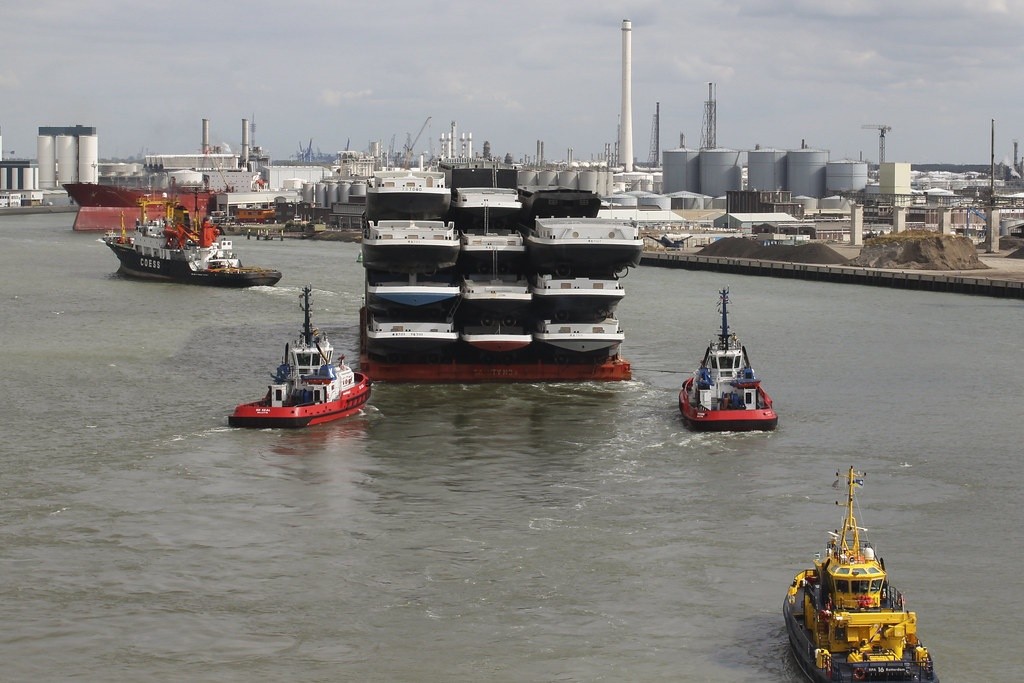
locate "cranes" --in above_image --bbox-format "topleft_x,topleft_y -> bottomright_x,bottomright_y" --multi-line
859,124 -> 892,164
386,133 -> 397,168
401,116 -> 433,169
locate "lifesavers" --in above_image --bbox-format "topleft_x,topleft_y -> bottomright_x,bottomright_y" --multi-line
853,667 -> 866,680
819,609 -> 826,619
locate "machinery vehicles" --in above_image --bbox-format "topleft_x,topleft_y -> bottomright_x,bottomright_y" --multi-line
964,206 -> 1002,239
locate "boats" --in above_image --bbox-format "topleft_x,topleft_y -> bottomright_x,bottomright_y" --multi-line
782,464 -> 941,683
61,154 -> 236,234
357,154 -> 647,385
678,284 -> 779,432
102,177 -> 283,289
226,280 -> 374,430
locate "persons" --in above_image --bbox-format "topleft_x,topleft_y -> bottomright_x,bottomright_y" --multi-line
732,332 -> 738,341
